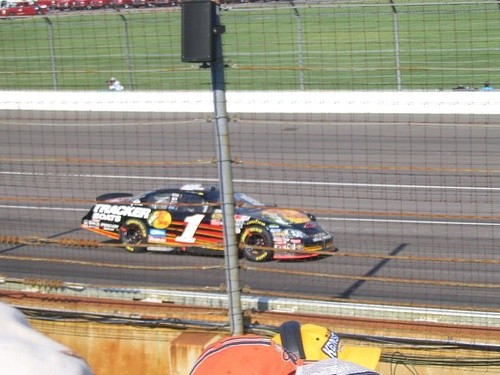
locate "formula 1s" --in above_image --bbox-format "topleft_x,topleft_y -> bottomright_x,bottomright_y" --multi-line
83,182 -> 337,264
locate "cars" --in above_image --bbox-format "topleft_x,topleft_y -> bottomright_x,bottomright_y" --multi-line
0,0 -> 184,18
